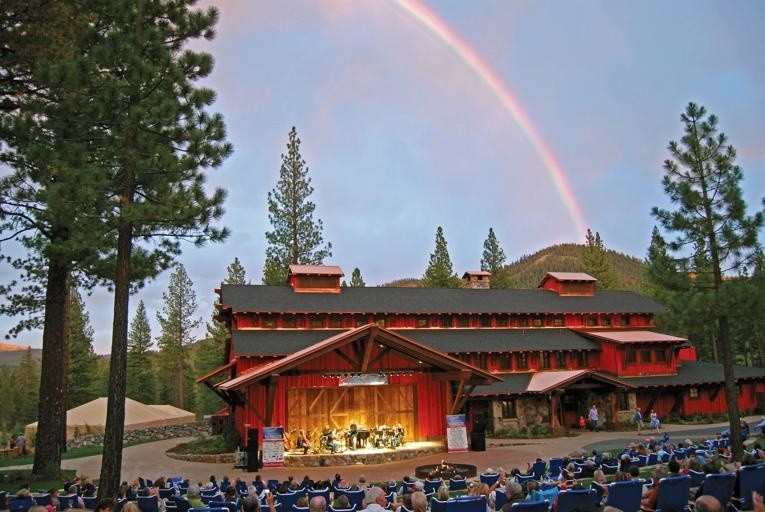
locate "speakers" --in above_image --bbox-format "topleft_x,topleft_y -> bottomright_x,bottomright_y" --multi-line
247,428 -> 258,472
471,413 -> 485,451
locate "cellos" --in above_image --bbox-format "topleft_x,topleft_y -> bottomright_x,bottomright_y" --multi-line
281,427 -> 294,450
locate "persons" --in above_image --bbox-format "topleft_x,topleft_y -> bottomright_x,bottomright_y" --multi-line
353,418 -> 765,512
588,404 -> 600,432
650,408 -> 661,434
635,407 -> 643,436
296,425 -> 335,456
10,432 -> 27,456
0,474 -> 351,512
